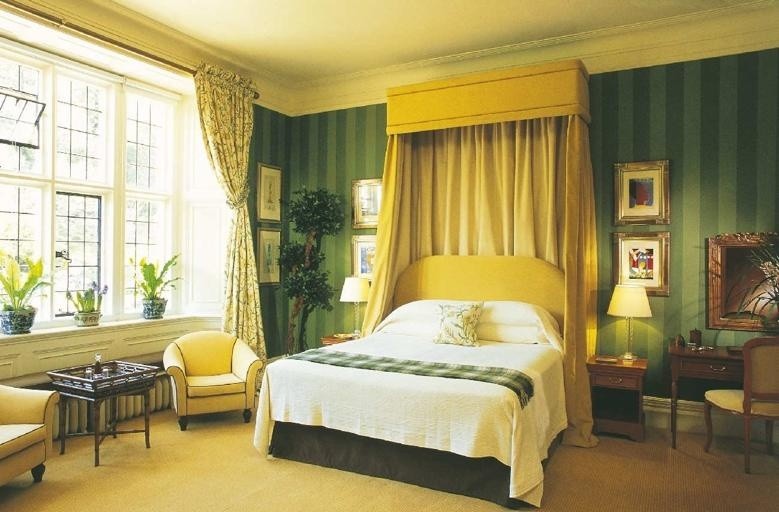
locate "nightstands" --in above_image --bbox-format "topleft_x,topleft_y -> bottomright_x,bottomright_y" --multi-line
320,336 -> 357,347
586,354 -> 646,443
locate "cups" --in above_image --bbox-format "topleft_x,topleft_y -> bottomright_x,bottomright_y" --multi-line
687,342 -> 698,351
85,367 -> 94,381
101,367 -> 110,380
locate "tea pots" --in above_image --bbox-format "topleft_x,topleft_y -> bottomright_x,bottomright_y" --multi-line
689,327 -> 702,347
674,334 -> 685,344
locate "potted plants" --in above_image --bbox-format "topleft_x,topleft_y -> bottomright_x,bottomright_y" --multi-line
65,288 -> 104,327
127,253 -> 183,319
0,249 -> 51,335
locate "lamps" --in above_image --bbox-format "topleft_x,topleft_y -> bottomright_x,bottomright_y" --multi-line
607,284 -> 653,363
337,276 -> 372,336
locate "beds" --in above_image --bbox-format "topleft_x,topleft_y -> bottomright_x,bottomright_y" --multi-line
254,254 -> 567,509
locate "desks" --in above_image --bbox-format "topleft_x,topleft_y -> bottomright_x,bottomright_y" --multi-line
45,359 -> 161,467
668,338 -> 779,449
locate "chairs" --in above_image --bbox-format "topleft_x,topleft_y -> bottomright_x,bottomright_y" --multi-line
704,340 -> 779,472
162,331 -> 263,432
1,383 -> 60,484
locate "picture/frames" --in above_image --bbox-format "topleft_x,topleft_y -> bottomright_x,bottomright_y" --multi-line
258,227 -> 281,287
613,160 -> 669,226
707,233 -> 779,332
350,177 -> 384,230
350,236 -> 383,281
258,161 -> 284,225
612,231 -> 670,299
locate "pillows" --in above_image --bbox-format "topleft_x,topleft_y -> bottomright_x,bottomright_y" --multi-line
435,301 -> 486,347
